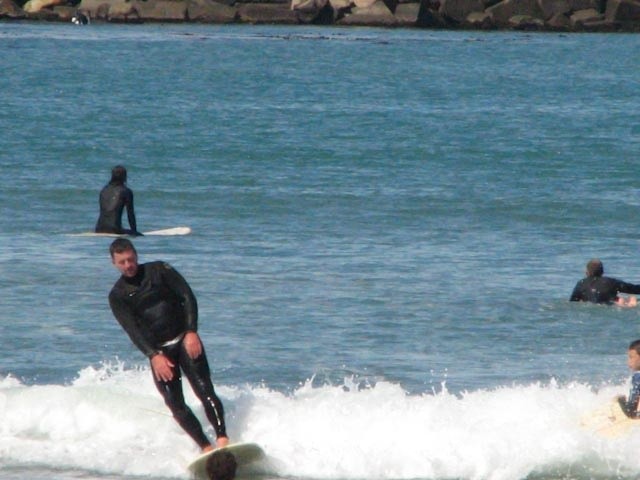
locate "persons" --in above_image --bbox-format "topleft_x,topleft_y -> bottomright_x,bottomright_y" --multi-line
569,258 -> 640,306
95,166 -> 144,237
108,238 -> 229,454
618,339 -> 640,419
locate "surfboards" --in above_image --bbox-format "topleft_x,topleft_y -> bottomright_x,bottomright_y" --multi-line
66,225 -> 192,238
188,440 -> 266,480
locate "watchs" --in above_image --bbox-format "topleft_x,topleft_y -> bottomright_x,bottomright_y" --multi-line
150,350 -> 163,359
